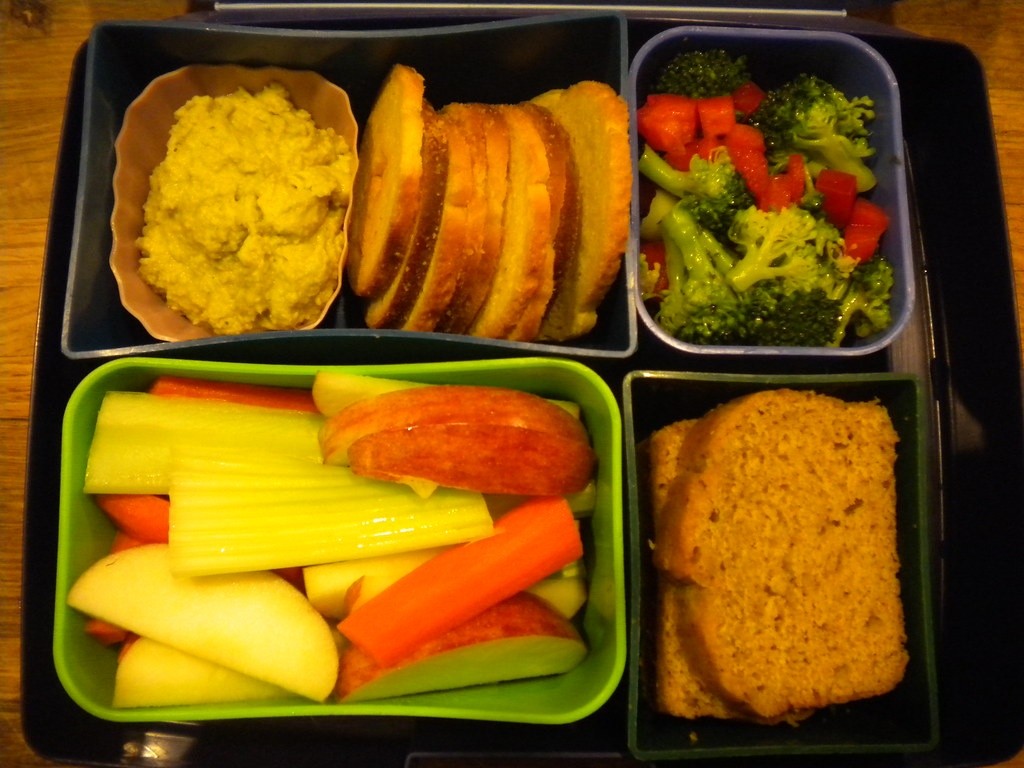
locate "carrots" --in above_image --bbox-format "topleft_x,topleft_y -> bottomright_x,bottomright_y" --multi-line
338,494 -> 584,666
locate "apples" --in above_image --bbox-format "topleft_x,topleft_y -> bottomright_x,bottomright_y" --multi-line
62,385 -> 601,712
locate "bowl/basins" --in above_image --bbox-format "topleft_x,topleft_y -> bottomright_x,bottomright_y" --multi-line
621,369 -> 943,761
60,11 -> 638,367
630,25 -> 914,355
53,357 -> 626,726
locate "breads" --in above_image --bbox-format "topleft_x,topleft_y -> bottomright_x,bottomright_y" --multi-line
651,390 -> 909,720
650,419 -> 817,726
357,55 -> 640,339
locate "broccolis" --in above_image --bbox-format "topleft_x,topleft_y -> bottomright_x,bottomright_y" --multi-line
634,47 -> 899,345
